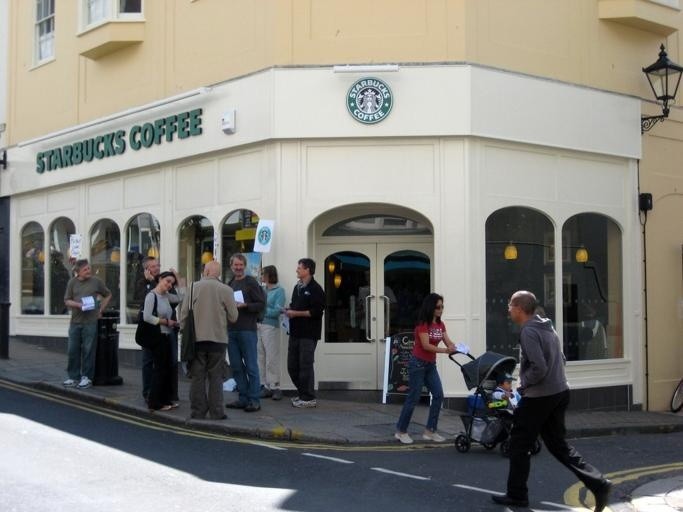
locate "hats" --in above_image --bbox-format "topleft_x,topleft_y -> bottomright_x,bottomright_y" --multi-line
497,370 -> 518,383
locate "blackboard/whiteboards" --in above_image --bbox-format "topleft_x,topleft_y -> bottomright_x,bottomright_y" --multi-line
385,333 -> 435,405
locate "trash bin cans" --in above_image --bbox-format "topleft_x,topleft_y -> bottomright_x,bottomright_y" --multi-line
95,317 -> 122,386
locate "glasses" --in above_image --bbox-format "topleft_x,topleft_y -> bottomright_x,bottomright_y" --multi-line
508,303 -> 517,307
149,264 -> 159,269
261,272 -> 267,275
435,305 -> 444,309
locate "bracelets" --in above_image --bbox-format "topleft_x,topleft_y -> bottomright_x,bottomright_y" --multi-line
166,318 -> 169,327
98,309 -> 104,314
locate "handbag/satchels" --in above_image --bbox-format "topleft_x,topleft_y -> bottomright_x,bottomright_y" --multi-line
179,282 -> 197,362
136,292 -> 163,351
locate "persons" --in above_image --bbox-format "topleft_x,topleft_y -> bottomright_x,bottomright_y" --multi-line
62,259 -> 112,391
224,252 -> 267,413
488,372 -> 528,445
394,293 -> 461,445
140,255 -> 177,402
135,273 -> 185,412
282,260 -> 325,409
491,289 -> 613,512
180,262 -> 238,421
254,264 -> 287,401
578,302 -> 609,360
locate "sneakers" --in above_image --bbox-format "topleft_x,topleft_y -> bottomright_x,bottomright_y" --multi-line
65,379 -> 81,386
245,401 -> 261,411
423,429 -> 446,443
260,387 -> 271,398
77,377 -> 94,389
220,414 -> 228,420
291,395 -> 298,400
493,494 -> 530,506
226,402 -> 241,409
270,390 -> 281,399
395,430 -> 413,443
292,399 -> 317,407
170,402 -> 179,407
590,481 -> 614,512
155,406 -> 172,410
